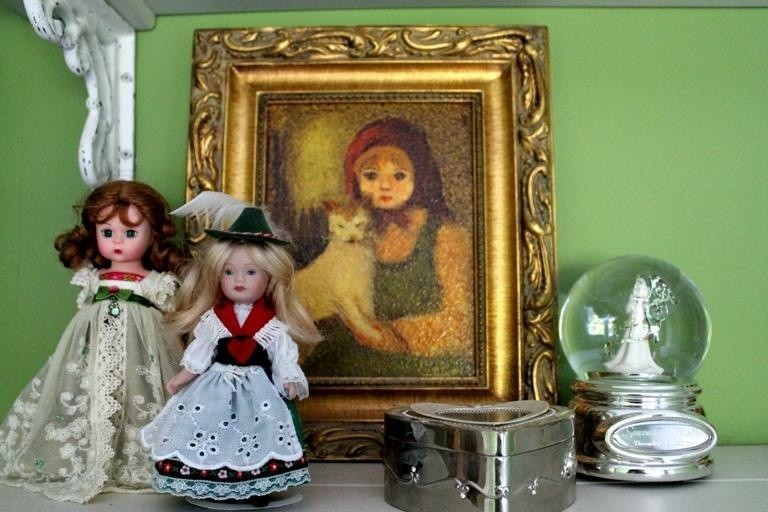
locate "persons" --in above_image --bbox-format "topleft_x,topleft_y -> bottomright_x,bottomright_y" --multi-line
315,117 -> 475,377
138,208 -> 326,510
0,181 -> 190,506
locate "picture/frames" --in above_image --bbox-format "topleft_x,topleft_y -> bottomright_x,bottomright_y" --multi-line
182,26 -> 560,461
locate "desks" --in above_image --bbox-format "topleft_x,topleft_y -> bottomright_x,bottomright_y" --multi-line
0,446 -> 768,511
